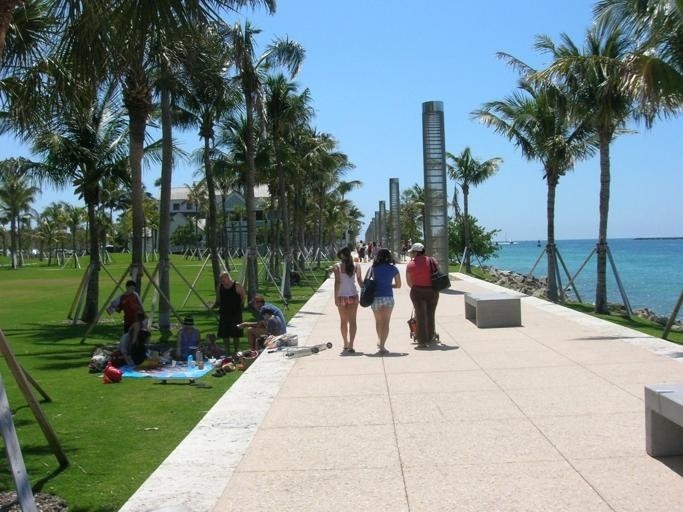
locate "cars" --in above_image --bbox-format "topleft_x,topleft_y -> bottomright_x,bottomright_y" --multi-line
0,243 -> 120,258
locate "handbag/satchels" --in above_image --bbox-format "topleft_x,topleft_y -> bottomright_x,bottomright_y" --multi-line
431,270 -> 451,292
360,278 -> 376,307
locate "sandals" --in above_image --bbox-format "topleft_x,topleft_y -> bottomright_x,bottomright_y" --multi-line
214,368 -> 226,377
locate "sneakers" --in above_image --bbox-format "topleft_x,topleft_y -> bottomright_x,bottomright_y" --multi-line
344,348 -> 355,354
376,344 -> 389,354
414,345 -> 427,350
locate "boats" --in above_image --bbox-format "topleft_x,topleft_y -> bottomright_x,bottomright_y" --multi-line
493,239 -> 521,245
536,239 -> 542,247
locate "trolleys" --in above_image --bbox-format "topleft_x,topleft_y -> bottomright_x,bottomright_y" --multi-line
406,302 -> 445,346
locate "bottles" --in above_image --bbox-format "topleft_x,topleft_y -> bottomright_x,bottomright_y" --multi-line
187,355 -> 193,371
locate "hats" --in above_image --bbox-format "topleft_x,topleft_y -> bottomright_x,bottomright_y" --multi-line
248,296 -> 264,304
183,315 -> 194,325
407,243 -> 425,253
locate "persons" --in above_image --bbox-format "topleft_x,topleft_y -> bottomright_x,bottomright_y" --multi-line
333,247 -> 363,352
363,249 -> 401,353
405,243 -> 442,347
113,269 -> 287,367
354,239 -> 411,262
